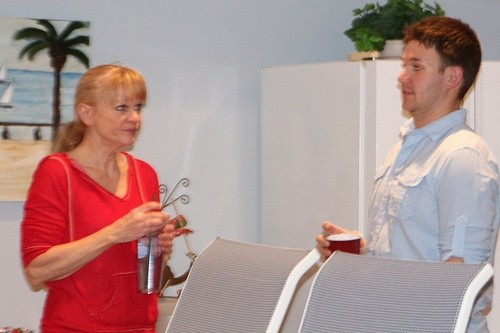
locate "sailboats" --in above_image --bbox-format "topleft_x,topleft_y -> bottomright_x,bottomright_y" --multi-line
0,81 -> 13,108
0,63 -> 7,80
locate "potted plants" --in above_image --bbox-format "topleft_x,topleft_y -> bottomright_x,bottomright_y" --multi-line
343,0 -> 445,58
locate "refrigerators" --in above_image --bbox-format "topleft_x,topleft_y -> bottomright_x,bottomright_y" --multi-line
260,61 -> 500,333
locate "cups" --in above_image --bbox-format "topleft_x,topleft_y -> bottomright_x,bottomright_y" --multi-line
326,234 -> 362,255
137,230 -> 164,294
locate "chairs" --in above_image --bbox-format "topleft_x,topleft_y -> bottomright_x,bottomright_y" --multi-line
164,238 -> 495,333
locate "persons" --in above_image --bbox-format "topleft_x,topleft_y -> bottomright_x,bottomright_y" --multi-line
315,16 -> 500,333
21,64 -> 173,333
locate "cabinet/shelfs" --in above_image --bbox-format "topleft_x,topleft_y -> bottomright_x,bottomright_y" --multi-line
259,58 -> 499,333
